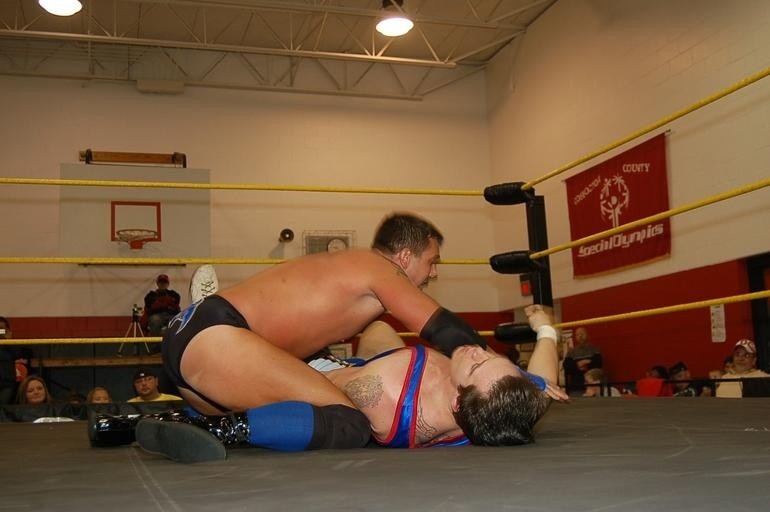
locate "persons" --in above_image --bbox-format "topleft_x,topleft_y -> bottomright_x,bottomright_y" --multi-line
299,304 -> 559,450
0,315 -> 183,405
561,323 -> 770,400
140,274 -> 181,356
85,214 -> 571,464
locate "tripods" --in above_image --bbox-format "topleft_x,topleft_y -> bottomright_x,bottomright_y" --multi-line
117,318 -> 151,355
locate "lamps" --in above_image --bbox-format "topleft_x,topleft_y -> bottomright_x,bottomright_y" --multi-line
375,0 -> 415,37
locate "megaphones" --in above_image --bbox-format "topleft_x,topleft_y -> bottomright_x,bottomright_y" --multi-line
279,228 -> 295,243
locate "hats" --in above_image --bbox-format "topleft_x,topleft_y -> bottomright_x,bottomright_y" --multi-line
132,368 -> 153,382
733,338 -> 756,354
158,274 -> 168,279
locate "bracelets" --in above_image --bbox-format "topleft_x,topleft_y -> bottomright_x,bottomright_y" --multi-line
536,325 -> 558,348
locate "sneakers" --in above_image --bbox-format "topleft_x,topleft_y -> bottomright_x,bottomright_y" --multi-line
189,263 -> 219,302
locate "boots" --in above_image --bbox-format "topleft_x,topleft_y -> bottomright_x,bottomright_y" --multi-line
134,409 -> 250,464
87,408 -> 186,444
